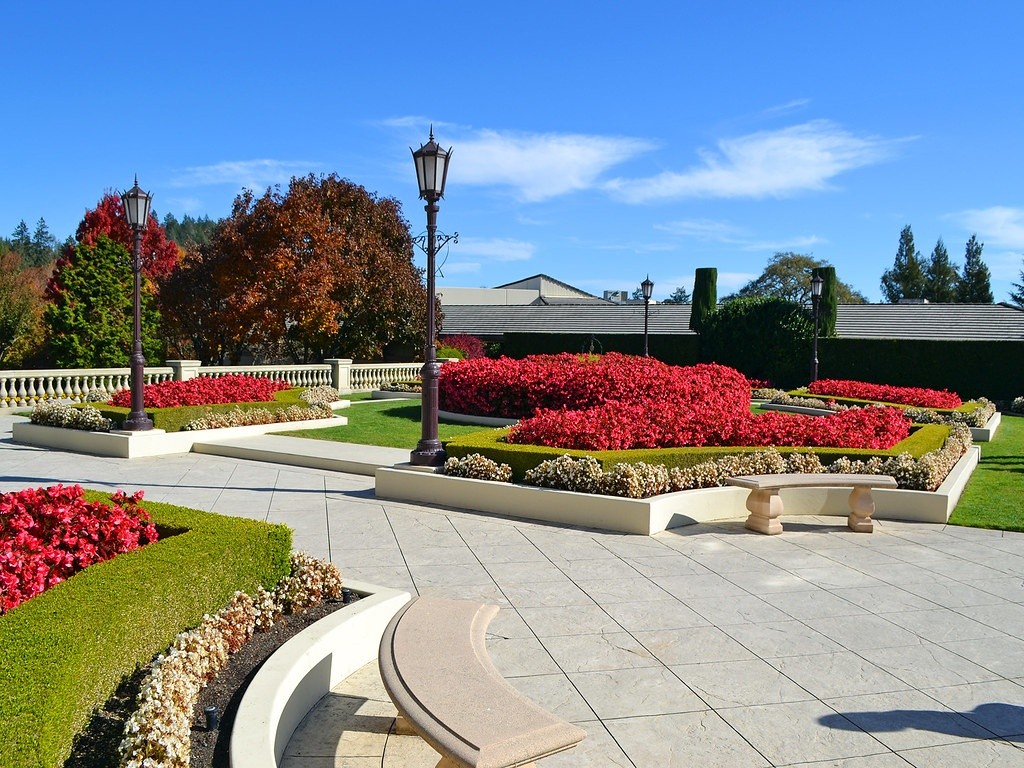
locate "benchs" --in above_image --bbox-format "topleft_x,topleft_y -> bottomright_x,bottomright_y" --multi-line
378,595 -> 587,768
728,474 -> 899,537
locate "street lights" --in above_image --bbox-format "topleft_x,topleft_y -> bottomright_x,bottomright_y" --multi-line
119,171 -> 155,432
408,122 -> 448,467
810,269 -> 824,382
640,273 -> 656,358
